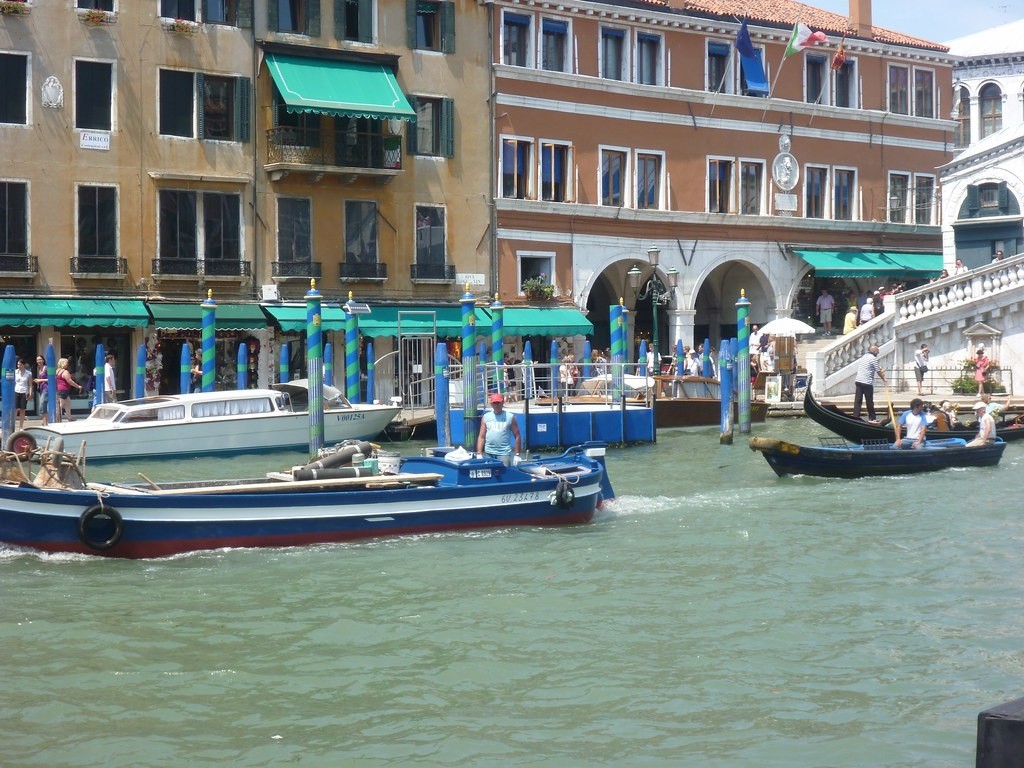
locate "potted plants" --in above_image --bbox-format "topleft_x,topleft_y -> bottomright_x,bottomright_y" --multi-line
950,375 -> 1006,396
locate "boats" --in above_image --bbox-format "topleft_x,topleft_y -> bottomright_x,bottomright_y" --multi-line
19,378 -> 403,462
803,373 -> 1024,446
581,374 -> 771,428
749,435 -> 1006,477
0,433 -> 615,558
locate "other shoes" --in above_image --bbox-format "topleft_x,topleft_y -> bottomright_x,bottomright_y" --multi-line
869,419 -> 881,422
976,393 -> 982,398
822,332 -> 832,336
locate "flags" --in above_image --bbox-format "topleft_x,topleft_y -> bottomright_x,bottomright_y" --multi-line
831,32 -> 847,72
734,17 -> 758,62
784,21 -> 829,60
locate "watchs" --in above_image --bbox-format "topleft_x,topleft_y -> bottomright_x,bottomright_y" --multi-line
515,452 -> 520,456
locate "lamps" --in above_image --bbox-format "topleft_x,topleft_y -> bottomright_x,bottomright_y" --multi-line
888,186 -> 942,212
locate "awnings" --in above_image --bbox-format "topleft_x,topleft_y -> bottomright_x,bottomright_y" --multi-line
0,298 -> 150,329
264,306 -> 594,336
146,302 -> 268,330
793,249 -> 905,280
882,250 -> 944,278
264,51 -> 417,124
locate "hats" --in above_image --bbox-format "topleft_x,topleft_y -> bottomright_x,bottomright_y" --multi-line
684,346 -> 690,352
971,402 -> 986,409
874,291 -> 881,296
689,349 -> 696,355
849,306 -> 858,311
490,393 -> 503,403
879,286 -> 885,290
976,350 -> 984,354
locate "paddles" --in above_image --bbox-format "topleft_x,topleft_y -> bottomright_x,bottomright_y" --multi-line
880,366 -> 899,440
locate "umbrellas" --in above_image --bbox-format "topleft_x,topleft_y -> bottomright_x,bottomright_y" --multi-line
757,317 -> 816,354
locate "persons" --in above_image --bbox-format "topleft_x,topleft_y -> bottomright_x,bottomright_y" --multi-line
915,345 -> 930,396
815,252 -> 1014,335
12,348 -> 203,429
477,394 -> 522,468
502,325 -> 766,401
973,349 -> 989,397
889,394 -> 1011,451
854,346 -> 886,422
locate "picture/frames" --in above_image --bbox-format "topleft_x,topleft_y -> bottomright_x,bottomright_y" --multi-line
765,376 -> 783,403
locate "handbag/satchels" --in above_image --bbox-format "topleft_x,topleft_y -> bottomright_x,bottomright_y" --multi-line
920,365 -> 929,373
86,376 -> 94,391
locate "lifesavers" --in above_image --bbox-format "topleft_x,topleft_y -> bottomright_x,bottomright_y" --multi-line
554,482 -> 575,509
77,504 -> 123,551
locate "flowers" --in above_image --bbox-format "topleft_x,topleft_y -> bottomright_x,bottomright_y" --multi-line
963,355 -> 999,369
0,0 -> 32,16
171,16 -> 198,39
79,7 -> 107,26
520,270 -> 557,291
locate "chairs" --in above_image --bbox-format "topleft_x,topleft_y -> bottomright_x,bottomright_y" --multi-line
933,411 -> 951,431
793,374 -> 808,400
817,435 -> 849,449
861,438 -> 890,450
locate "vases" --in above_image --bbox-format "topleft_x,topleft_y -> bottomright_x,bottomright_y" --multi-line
964,365 -> 969,369
970,364 -> 974,369
992,367 -> 997,369
524,290 -> 549,301
